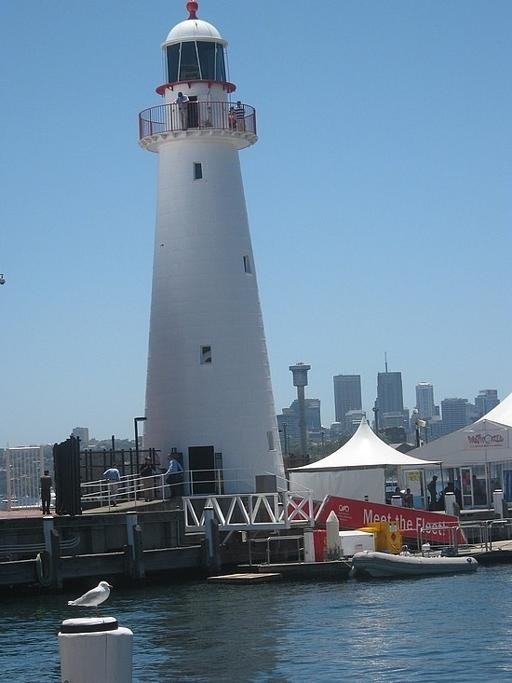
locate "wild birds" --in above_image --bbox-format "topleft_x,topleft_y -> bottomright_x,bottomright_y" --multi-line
66,580 -> 113,618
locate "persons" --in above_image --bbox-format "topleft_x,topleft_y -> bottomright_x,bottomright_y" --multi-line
394,486 -> 402,496
232,100 -> 246,131
139,455 -> 158,501
173,91 -> 191,130
403,488 -> 413,506
102,462 -> 122,506
39,469 -> 55,515
162,453 -> 184,498
227,107 -> 236,128
427,473 -> 438,504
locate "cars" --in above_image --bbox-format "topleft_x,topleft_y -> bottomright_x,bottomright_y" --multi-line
386,480 -> 399,495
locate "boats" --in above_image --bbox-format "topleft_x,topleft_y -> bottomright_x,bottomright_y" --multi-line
350,548 -> 480,582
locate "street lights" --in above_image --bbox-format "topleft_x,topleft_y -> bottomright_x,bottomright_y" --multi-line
412,408 -> 427,446
373,406 -> 379,437
133,414 -> 150,499
282,421 -> 289,456
